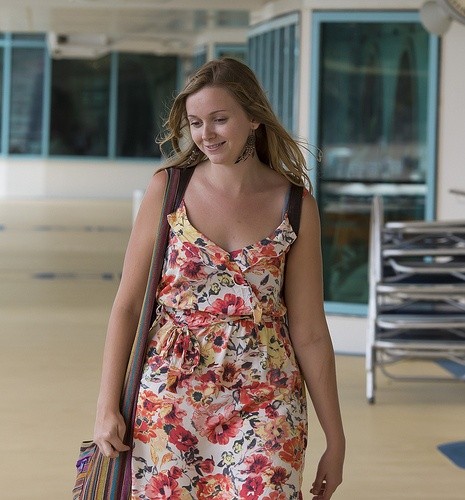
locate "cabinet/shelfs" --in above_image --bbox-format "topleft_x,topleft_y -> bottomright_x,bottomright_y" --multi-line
365,194 -> 465,404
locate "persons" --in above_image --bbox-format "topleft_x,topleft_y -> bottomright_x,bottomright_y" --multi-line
92,58 -> 348,499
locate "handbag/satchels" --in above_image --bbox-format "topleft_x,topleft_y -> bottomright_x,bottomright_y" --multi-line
71,437 -> 132,500
